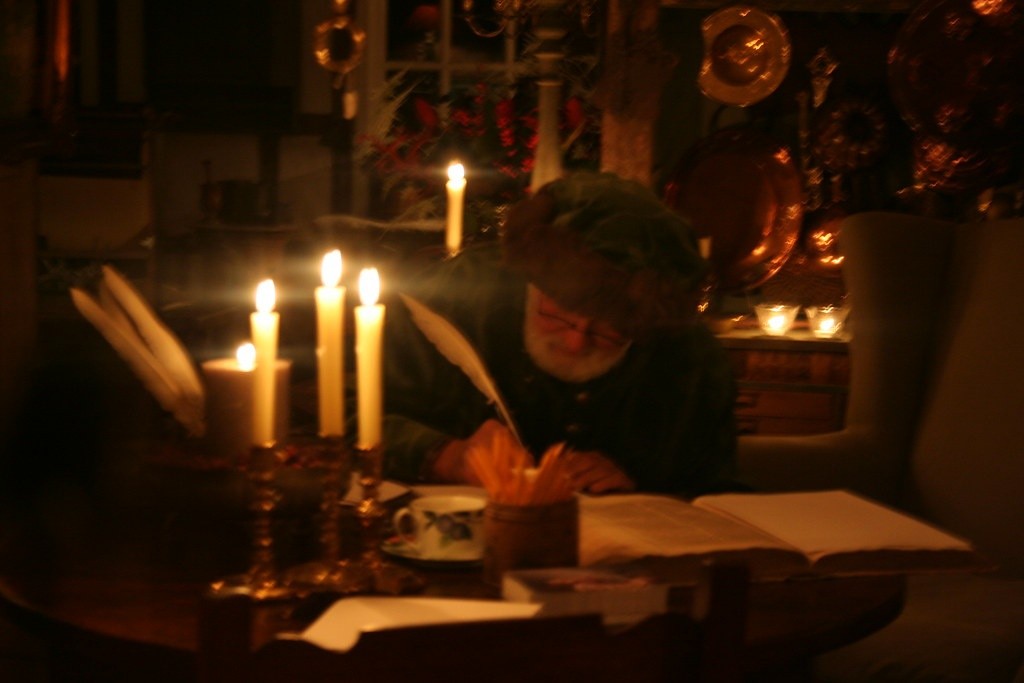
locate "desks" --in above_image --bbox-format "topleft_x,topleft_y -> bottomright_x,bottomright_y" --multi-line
0,471 -> 909,683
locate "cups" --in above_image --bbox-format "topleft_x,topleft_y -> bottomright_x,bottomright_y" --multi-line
754,304 -> 800,335
484,496 -> 578,600
804,306 -> 849,339
392,495 -> 486,559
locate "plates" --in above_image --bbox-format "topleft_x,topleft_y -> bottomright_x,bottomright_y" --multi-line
382,532 -> 485,563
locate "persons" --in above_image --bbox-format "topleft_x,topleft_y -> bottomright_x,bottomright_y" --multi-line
347,172 -> 738,496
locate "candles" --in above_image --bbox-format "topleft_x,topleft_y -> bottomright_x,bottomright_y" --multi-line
441,159 -> 468,257
309,247 -> 351,437
246,274 -> 283,449
352,258 -> 389,450
201,339 -> 294,458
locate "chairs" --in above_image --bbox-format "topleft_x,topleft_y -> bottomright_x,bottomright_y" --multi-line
734,212 -> 1023,683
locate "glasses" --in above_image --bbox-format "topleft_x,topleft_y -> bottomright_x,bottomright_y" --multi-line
534,295 -> 627,355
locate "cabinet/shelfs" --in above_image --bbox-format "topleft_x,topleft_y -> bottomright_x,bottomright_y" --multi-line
717,318 -> 850,437
